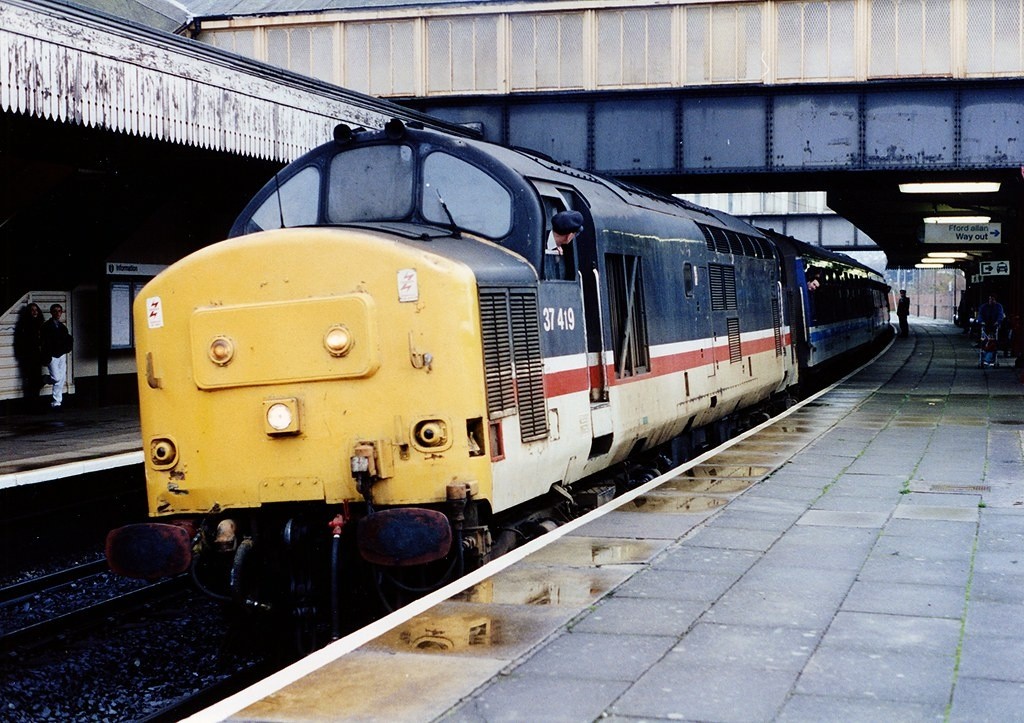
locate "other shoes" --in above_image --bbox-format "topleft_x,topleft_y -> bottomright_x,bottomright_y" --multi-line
40,375 -> 49,389
52,405 -> 63,416
983,362 -> 994,366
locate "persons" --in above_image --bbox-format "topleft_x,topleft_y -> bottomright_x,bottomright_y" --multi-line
806,277 -> 820,291
978,296 -> 1003,367
897,290 -> 910,336
544,211 -> 584,280
13,303 -> 73,408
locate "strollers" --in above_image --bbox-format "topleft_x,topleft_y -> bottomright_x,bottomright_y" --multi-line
978,321 -> 1001,369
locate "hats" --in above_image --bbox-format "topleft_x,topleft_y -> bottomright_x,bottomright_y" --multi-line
551,210 -> 583,232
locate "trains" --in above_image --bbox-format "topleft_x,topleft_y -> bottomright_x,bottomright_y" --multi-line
101,126 -> 894,595
